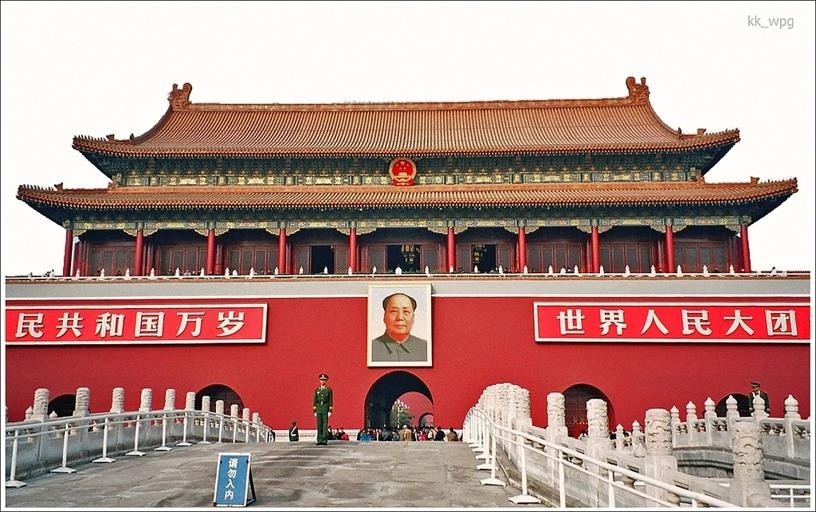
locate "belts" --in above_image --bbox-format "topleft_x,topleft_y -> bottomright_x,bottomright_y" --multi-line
319,401 -> 326,405
291,434 -> 296,436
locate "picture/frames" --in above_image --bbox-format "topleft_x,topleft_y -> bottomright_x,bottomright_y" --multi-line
367,282 -> 432,368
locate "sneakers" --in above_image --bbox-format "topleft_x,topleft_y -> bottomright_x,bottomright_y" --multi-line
315,443 -> 325,445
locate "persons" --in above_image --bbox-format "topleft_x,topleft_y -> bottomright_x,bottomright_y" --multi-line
287,420 -> 299,442
309,373 -> 334,445
370,292 -> 427,363
327,423 -> 349,441
748,380 -> 772,418
356,422 -> 463,442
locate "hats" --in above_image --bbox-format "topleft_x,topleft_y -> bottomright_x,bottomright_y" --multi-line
292,422 -> 296,425
319,373 -> 329,380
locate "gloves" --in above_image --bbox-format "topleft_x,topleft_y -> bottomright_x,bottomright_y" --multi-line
314,412 -> 317,417
328,411 -> 332,418
